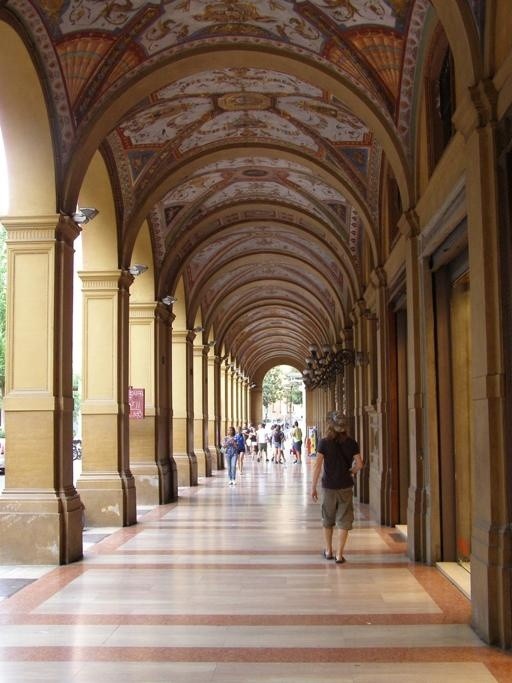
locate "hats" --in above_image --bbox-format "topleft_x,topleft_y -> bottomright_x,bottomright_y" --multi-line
328,409 -> 345,432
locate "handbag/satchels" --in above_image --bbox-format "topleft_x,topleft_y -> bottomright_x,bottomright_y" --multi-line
219,445 -> 228,454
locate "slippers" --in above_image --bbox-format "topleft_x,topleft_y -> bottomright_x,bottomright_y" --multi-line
335,555 -> 346,563
323,548 -> 332,559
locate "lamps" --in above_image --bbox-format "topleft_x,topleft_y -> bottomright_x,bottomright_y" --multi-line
298,338 -> 358,393
73,205 -> 258,391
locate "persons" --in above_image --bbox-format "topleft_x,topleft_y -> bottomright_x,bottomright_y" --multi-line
223,427 -> 240,484
234,427 -> 246,474
310,412 -> 362,563
241,422 -> 302,464
